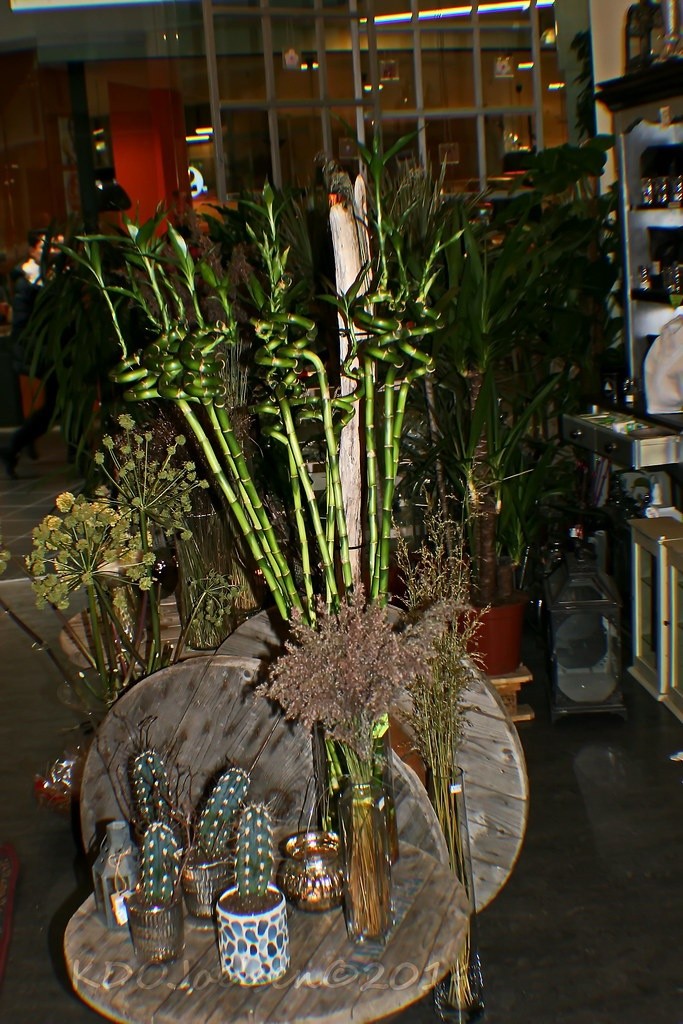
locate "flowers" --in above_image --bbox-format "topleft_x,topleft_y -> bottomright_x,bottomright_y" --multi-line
1,103 -> 471,716
250,577 -> 475,936
386,463 -> 495,1013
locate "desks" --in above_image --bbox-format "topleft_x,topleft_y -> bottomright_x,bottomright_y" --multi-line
63,824 -> 471,1024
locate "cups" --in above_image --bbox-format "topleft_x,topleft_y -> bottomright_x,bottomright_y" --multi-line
630,173 -> 683,304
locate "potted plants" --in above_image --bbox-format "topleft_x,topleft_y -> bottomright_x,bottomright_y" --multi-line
128,741 -> 192,853
363,131 -> 634,678
168,768 -> 250,920
214,795 -> 290,987
123,822 -> 189,968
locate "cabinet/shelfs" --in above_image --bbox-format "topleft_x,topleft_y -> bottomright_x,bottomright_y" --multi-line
557,55 -> 683,725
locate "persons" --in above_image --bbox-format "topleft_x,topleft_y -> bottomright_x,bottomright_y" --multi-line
0,219 -> 76,477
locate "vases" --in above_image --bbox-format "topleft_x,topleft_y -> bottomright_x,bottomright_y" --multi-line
168,508 -> 239,652
424,766 -> 489,1022
337,781 -> 392,948
55,660 -> 166,832
308,715 -> 401,865
